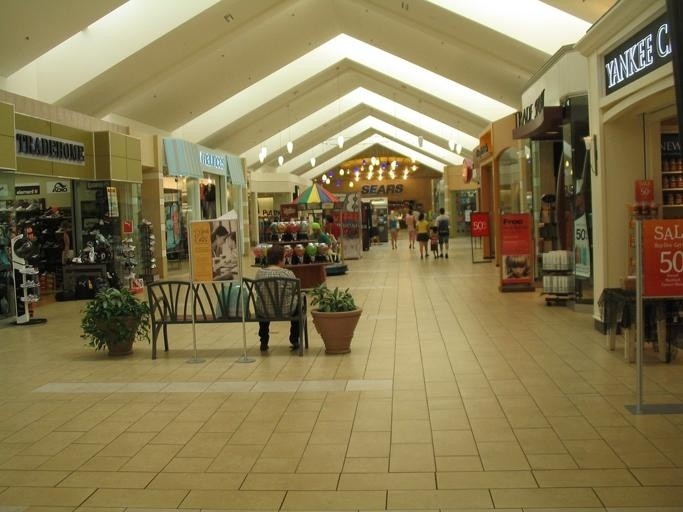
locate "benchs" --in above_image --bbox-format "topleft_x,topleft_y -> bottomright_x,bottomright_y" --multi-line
147,278 -> 308,360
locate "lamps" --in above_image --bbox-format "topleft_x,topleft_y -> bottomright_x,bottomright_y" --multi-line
257,65 -> 463,185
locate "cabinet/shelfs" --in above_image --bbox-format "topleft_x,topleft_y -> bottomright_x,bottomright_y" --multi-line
0,197 -> 122,326
116,220 -> 144,294
541,268 -> 577,306
597,288 -> 683,363
626,203 -> 662,279
645,113 -> 683,220
137,224 -> 161,283
251,218 -> 330,290
389,200 -> 424,226
164,187 -> 183,260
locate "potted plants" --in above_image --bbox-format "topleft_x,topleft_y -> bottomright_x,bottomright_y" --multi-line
78,282 -> 152,355
305,282 -> 363,355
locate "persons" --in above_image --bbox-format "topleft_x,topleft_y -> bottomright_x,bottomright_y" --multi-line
254,245 -> 304,351
321,216 -> 340,241
389,210 -> 400,250
435,207 -> 450,258
211,225 -> 239,281
430,227 -> 440,258
416,212 -> 430,258
405,209 -> 417,248
